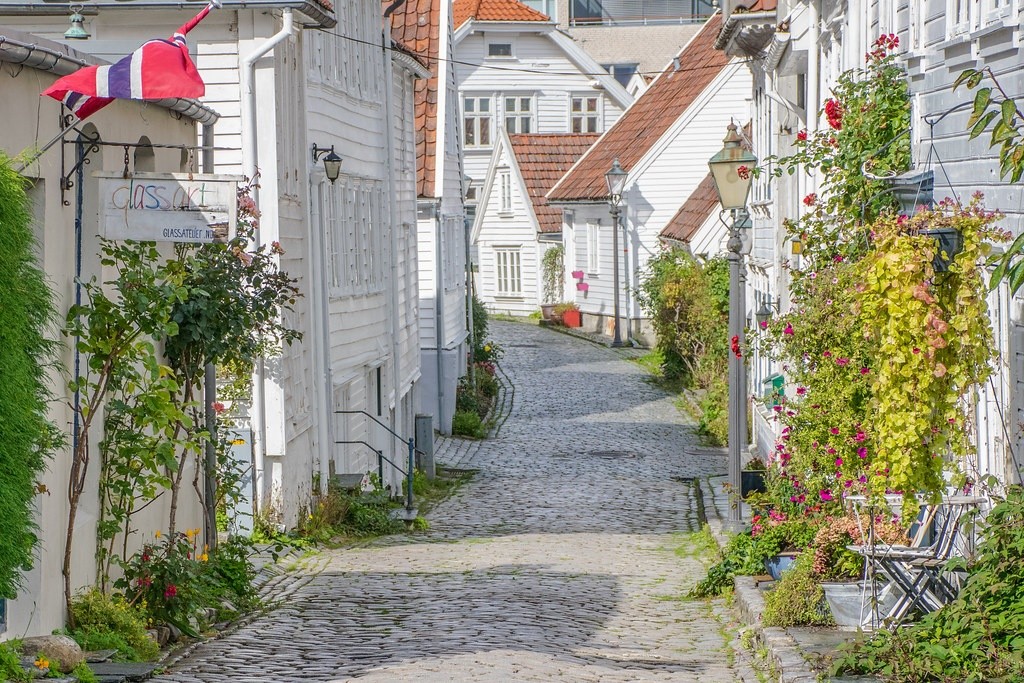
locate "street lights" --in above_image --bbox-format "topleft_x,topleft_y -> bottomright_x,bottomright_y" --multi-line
707,115 -> 761,539
605,156 -> 630,348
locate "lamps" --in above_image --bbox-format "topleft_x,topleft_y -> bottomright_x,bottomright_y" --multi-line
311,143 -> 343,185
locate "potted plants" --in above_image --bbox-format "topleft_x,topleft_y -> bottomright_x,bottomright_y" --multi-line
539,244 -> 581,328
691,471 -> 915,637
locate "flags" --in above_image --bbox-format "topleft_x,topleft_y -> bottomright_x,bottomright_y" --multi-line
39,4 -> 210,122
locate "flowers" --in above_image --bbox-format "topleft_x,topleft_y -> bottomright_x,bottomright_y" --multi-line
852,193 -> 1016,536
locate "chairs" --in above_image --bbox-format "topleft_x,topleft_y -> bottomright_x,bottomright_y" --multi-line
845,470 -> 987,632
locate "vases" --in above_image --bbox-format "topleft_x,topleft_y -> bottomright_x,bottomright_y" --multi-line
919,225 -> 965,273
